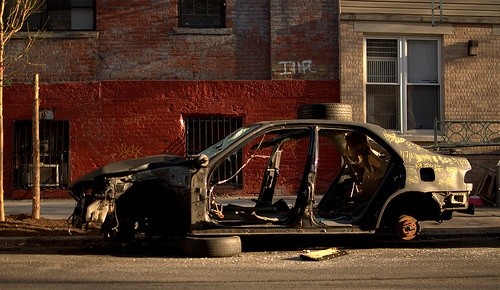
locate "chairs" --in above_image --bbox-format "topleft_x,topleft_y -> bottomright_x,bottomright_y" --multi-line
214,142 -> 284,216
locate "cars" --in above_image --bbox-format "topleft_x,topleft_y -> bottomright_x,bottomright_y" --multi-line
66,119 -> 474,258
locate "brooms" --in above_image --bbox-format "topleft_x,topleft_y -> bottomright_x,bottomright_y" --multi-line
469,175 -> 485,206
